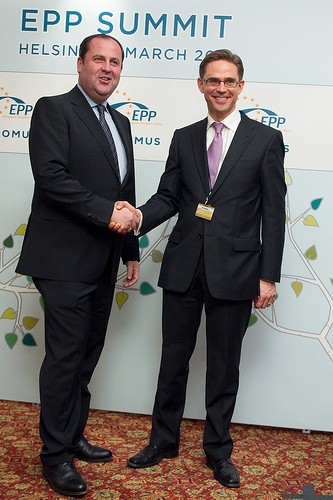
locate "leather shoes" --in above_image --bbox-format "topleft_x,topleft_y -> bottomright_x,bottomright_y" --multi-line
206,453 -> 240,488
126,443 -> 180,468
75,434 -> 112,464
42,460 -> 88,497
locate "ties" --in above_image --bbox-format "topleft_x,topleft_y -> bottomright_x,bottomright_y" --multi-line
206,122 -> 225,188
95,104 -> 121,187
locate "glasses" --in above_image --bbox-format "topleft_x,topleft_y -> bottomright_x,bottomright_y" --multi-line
200,75 -> 243,90
